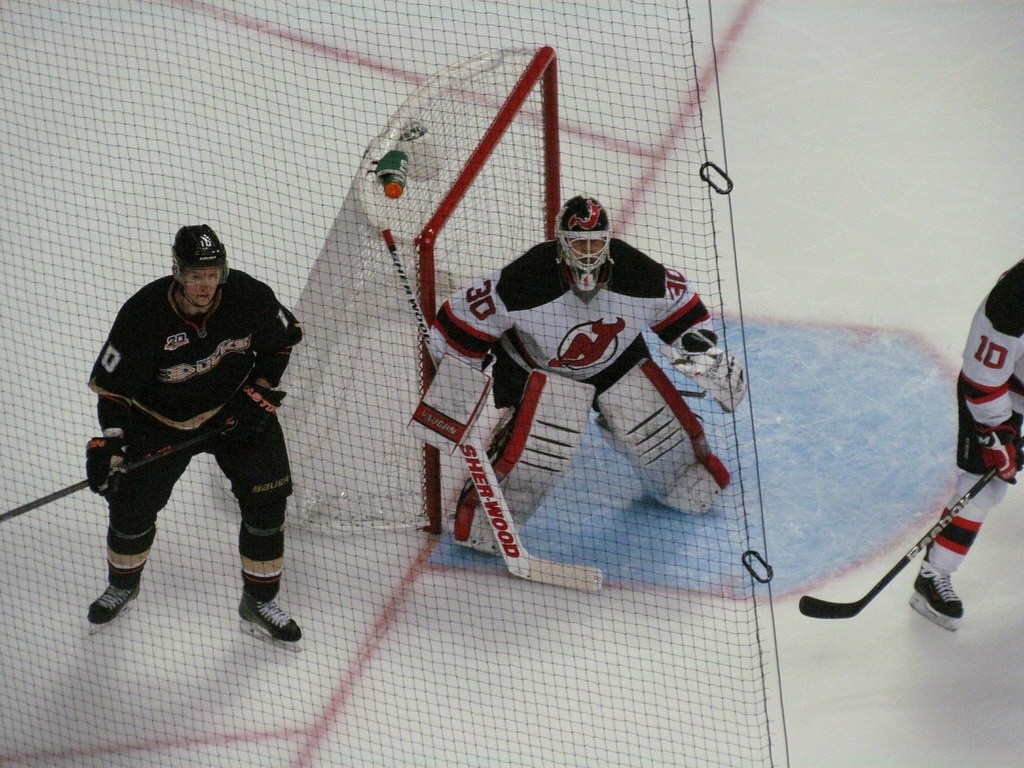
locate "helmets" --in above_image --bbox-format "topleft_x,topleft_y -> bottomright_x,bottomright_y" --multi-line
172,224 -> 227,269
553,194 -> 613,292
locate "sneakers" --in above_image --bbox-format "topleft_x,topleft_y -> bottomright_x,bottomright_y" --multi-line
238,592 -> 303,654
909,557 -> 964,630
87,581 -> 140,635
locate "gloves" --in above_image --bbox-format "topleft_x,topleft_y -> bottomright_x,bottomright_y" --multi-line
85,437 -> 127,499
974,423 -> 1024,485
212,381 -> 287,456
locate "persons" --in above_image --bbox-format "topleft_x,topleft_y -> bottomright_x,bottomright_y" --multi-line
85,225 -> 303,643
913,257 -> 1024,619
430,194 -> 747,557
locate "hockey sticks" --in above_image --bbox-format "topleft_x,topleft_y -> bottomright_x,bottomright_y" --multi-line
0,422 -> 238,524
797,436 -> 1024,621
379,224 -> 604,594
